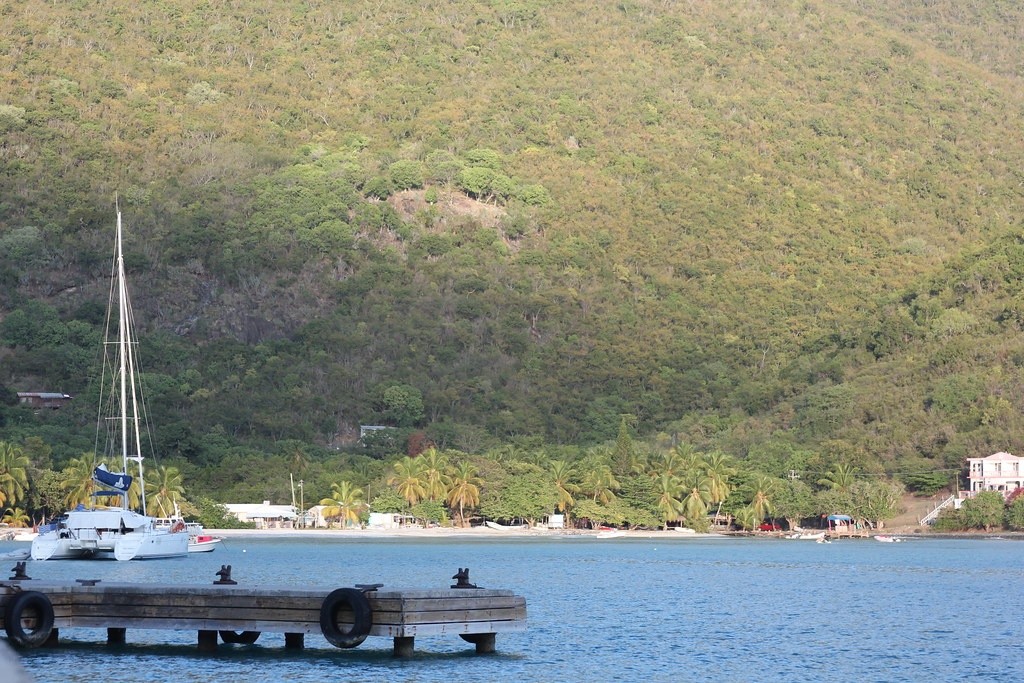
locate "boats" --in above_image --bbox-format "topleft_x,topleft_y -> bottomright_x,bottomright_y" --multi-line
485,521 -> 526,530
873,536 -> 902,543
0,549 -> 31,561
674,527 -> 696,533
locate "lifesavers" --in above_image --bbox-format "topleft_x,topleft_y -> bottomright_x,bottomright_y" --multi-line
221,629 -> 262,645
4,590 -> 56,653
320,588 -> 372,651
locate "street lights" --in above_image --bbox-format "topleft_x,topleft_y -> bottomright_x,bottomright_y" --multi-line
298,478 -> 304,531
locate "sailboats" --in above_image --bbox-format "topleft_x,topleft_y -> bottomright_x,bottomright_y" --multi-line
29,207 -> 223,565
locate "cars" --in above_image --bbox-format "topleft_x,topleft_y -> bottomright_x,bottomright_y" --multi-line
757,521 -> 780,531
